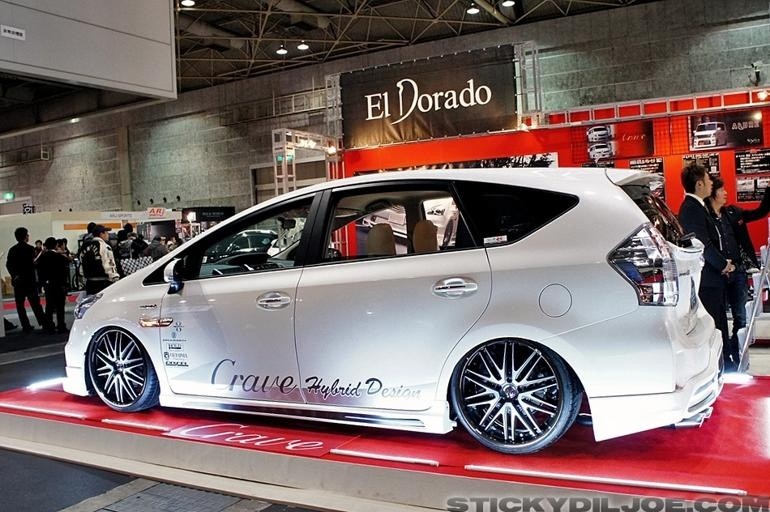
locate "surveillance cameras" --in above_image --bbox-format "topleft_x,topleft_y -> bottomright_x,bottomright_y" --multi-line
752,59 -> 762,69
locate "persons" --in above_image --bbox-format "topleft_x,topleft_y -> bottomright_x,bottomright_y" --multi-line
677,164 -> 733,373
704,175 -> 770,369
3,210 -> 225,333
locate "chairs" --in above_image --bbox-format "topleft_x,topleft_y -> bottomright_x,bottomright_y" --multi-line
366,223 -> 396,257
413,219 -> 437,252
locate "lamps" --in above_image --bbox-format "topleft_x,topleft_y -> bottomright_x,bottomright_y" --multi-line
276,40 -> 288,54
467,2 -> 480,14
296,41 -> 309,50
502,0 -> 516,7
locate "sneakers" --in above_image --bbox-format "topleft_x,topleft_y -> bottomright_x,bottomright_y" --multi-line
5,323 -> 70,335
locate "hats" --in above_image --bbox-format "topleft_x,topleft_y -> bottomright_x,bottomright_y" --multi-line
92,224 -> 112,236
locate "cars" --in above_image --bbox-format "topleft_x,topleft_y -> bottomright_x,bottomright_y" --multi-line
362,197 -> 459,247
61,169 -> 725,454
202,230 -> 277,264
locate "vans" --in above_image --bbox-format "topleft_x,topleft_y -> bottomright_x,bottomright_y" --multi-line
693,122 -> 729,147
588,124 -> 609,141
590,143 -> 612,159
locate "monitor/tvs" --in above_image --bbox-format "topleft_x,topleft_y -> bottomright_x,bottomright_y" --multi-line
146,220 -> 177,241
